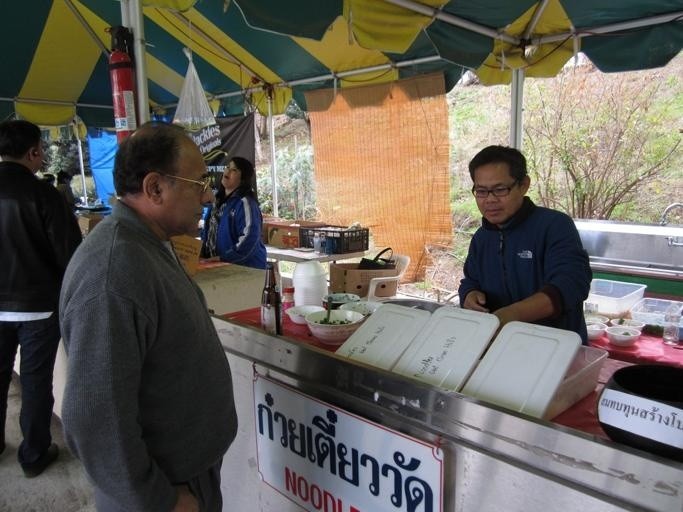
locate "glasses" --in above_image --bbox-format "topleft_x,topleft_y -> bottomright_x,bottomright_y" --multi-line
471,173 -> 523,198
150,163 -> 213,195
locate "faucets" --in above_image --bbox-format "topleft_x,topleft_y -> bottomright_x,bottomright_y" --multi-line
659,203 -> 683,226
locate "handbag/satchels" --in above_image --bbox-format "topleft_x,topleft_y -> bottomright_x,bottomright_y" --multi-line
358,247 -> 398,270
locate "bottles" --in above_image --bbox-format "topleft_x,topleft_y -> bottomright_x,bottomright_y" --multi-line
662,302 -> 681,346
260,261 -> 281,335
281,287 -> 295,327
313,232 -> 321,255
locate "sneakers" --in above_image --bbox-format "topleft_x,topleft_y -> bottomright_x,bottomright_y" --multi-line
18,441 -> 61,479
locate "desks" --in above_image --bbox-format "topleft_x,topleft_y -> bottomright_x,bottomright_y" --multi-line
264,246 -> 370,295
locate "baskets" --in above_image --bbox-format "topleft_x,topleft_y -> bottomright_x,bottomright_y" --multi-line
298,224 -> 370,255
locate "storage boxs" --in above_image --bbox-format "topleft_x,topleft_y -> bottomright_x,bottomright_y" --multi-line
261,219 -> 287,245
78,215 -> 104,235
583,278 -> 647,316
268,222 -> 299,249
629,298 -> 683,324
527,345 -> 608,421
330,264 -> 397,297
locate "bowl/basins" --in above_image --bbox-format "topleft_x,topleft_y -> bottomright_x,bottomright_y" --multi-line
606,326 -> 641,347
306,310 -> 365,346
609,319 -> 646,332
337,301 -> 383,317
321,293 -> 360,309
586,321 -> 607,342
321,335 -> 326,336
586,313 -> 610,324
285,305 -> 326,325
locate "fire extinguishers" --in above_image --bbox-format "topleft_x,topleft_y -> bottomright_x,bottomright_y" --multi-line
103,25 -> 139,145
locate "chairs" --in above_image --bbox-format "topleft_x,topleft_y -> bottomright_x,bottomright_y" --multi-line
368,254 -> 410,300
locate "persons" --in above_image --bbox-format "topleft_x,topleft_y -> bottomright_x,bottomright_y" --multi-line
56,120 -> 238,512
0,121 -> 84,477
458,144 -> 593,346
43,169 -> 82,211
201,158 -> 267,269
